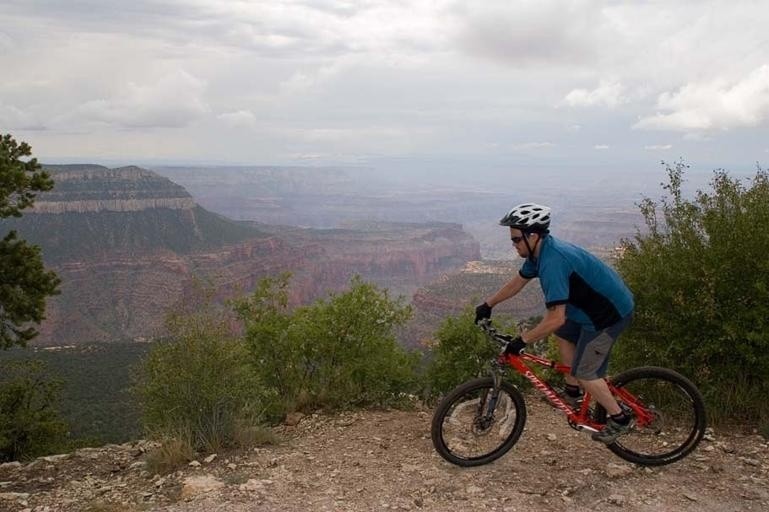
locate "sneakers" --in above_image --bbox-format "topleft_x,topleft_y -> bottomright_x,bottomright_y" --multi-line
541,391 -> 584,411
592,419 -> 636,442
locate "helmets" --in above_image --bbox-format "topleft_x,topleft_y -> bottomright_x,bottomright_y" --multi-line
499,203 -> 552,232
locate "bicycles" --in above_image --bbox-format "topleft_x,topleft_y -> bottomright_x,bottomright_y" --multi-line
431,316 -> 707,467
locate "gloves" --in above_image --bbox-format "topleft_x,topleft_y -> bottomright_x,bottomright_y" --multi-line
475,302 -> 491,324
504,337 -> 527,356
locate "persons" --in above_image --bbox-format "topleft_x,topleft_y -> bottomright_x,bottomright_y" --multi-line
475,204 -> 635,444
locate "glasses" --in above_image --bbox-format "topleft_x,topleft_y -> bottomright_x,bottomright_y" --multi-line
511,237 -> 525,243
